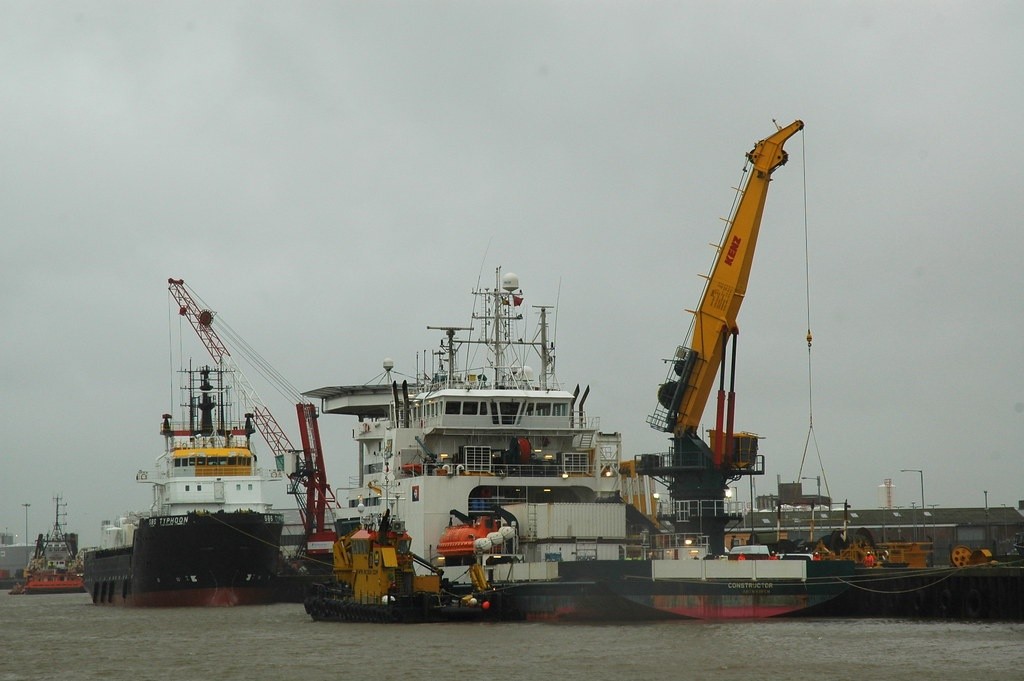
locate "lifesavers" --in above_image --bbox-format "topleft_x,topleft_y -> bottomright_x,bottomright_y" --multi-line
271,471 -> 278,478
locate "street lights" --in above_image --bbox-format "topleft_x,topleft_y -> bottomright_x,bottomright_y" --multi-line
1001,503 -> 1007,540
984,490 -> 989,548
844,504 -> 852,543
929,504 -> 940,565
901,469 -> 927,541
22,502 -> 31,566
880,506 -> 890,543
910,501 -> 921,541
894,506 -> 904,541
802,476 -> 822,539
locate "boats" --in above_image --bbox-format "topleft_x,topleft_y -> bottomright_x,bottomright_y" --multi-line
7,584 -> 25,593
164,120 -> 1024,624
24,494 -> 85,594
80,356 -> 285,608
